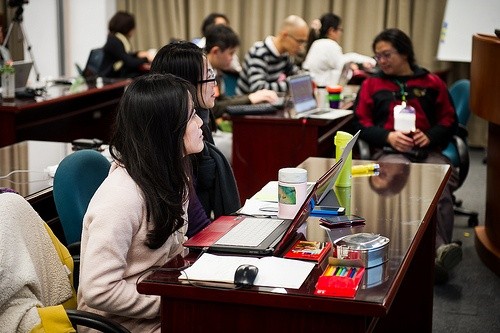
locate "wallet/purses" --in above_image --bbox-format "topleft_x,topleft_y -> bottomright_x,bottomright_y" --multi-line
225,102 -> 276,118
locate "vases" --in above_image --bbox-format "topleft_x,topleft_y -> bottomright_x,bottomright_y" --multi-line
1,72 -> 16,103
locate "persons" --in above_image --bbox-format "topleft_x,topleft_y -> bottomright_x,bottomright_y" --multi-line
352,28 -> 463,274
302,13 -> 377,88
74,74 -> 204,333
199,24 -> 280,120
81,12 -> 155,81
146,40 -> 242,240
234,14 -> 310,96
196,13 -> 242,72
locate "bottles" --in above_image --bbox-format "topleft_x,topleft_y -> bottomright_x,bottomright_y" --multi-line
278,167 -> 307,220
333,187 -> 352,216
333,130 -> 353,187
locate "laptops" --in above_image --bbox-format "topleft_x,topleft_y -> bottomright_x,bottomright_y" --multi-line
188,130 -> 362,255
269,74 -> 354,120
9,62 -> 33,89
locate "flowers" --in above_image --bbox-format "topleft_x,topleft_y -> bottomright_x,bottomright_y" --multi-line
0,59 -> 16,74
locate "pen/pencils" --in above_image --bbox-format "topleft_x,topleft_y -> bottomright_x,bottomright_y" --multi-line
294,241 -> 316,255
325,266 -> 357,279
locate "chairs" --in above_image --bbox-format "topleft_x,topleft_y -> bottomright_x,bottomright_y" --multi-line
0,151 -> 131,333
347,78 -> 479,226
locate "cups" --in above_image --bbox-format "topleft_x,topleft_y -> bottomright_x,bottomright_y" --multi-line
326,85 -> 341,108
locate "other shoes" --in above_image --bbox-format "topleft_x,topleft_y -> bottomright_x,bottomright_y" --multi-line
433,243 -> 463,276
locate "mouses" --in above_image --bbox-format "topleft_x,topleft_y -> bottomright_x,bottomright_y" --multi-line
233,264 -> 259,287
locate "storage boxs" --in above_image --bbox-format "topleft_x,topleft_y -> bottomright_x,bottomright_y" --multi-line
313,257 -> 367,298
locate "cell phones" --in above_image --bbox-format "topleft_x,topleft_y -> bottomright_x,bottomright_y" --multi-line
311,206 -> 346,215
320,214 -> 366,225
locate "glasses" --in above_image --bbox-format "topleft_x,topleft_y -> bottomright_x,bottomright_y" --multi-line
335,28 -> 344,33
288,35 -> 308,45
198,68 -> 216,85
373,50 -> 397,61
375,169 -> 391,180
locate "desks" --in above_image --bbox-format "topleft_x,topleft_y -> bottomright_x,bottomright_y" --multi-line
132,156 -> 453,333
222,82 -> 359,207
0,139 -> 120,246
0,74 -> 134,148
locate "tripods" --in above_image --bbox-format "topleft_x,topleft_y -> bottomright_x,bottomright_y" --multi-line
2,6 -> 39,80
